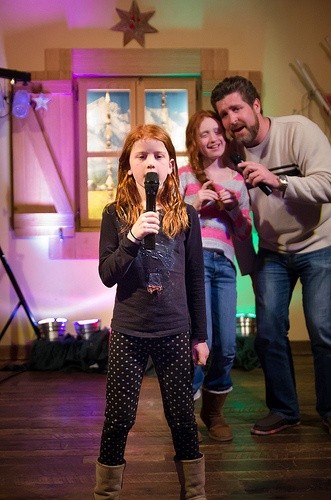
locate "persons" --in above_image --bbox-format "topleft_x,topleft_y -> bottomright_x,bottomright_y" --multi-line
175,110 -> 252,443
210,75 -> 331,442
95,125 -> 209,500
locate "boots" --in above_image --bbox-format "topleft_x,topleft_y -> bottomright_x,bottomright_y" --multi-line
197,431 -> 202,442
94,459 -> 126,500
173,452 -> 207,500
200,387 -> 233,441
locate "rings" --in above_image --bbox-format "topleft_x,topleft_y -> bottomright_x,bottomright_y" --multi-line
280,174 -> 289,195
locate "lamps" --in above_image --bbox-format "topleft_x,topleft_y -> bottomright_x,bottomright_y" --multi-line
12,90 -> 30,118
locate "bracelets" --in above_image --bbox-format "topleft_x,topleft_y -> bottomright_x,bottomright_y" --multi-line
129,228 -> 143,242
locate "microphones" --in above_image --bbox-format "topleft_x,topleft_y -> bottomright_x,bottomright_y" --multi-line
143,172 -> 159,249
230,151 -> 273,196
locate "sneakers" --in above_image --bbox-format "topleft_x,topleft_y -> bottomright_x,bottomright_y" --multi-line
252,412 -> 301,434
322,416 -> 331,434
